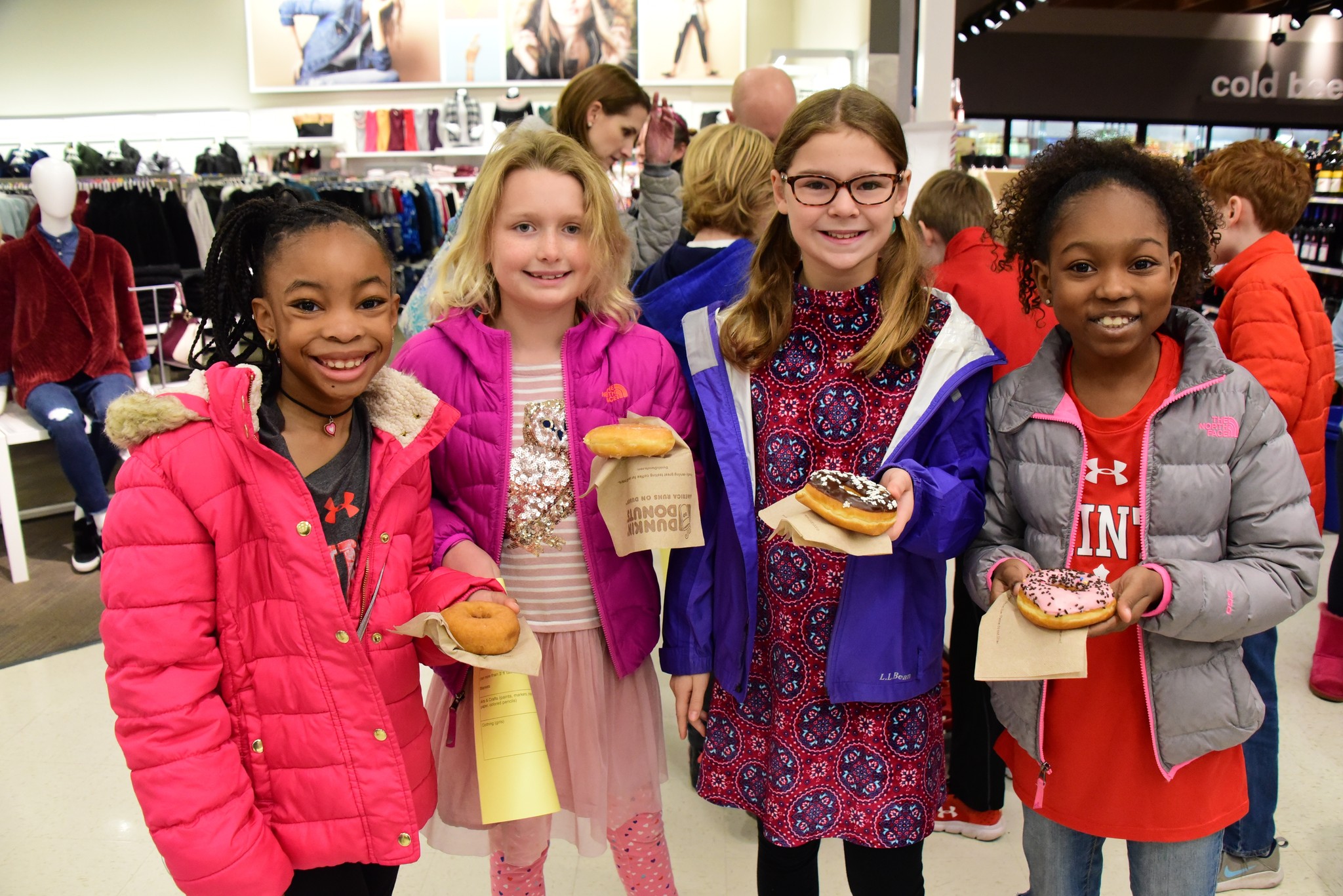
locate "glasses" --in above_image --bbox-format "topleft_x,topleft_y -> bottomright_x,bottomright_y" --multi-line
780,173 -> 903,207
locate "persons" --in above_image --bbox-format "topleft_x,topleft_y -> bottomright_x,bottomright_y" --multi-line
102,186 -> 519,896
277,1 -> 719,86
396,66 -> 798,353
393,127 -> 707,896
664,86 -> 1008,896
932,137 -> 1343,895
0,157 -> 150,572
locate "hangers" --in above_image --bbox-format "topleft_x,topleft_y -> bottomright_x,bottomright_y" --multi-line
0,178 -> 247,202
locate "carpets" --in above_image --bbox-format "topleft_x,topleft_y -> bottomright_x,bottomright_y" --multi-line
0,424 -> 122,670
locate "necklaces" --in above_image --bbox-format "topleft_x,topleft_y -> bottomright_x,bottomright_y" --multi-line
280,387 -> 356,437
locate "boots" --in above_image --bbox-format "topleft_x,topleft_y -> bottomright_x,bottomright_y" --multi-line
1308,602 -> 1343,704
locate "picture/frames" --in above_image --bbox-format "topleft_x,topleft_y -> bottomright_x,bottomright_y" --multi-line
245,0 -> 749,94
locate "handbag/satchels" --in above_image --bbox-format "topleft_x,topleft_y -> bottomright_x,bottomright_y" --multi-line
294,113 -> 335,136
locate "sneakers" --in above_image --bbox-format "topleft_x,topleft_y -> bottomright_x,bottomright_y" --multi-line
70,515 -> 106,572
932,781 -> 1005,842
1214,832 -> 1289,892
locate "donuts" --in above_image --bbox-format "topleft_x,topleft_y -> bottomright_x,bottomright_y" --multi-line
1017,568 -> 1116,629
795,469 -> 898,536
583,423 -> 675,459
440,601 -> 520,654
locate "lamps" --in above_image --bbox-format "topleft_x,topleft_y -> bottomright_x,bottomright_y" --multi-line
957,0 -> 1343,46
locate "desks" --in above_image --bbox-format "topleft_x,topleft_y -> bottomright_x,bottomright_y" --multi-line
0,381 -> 188,583
